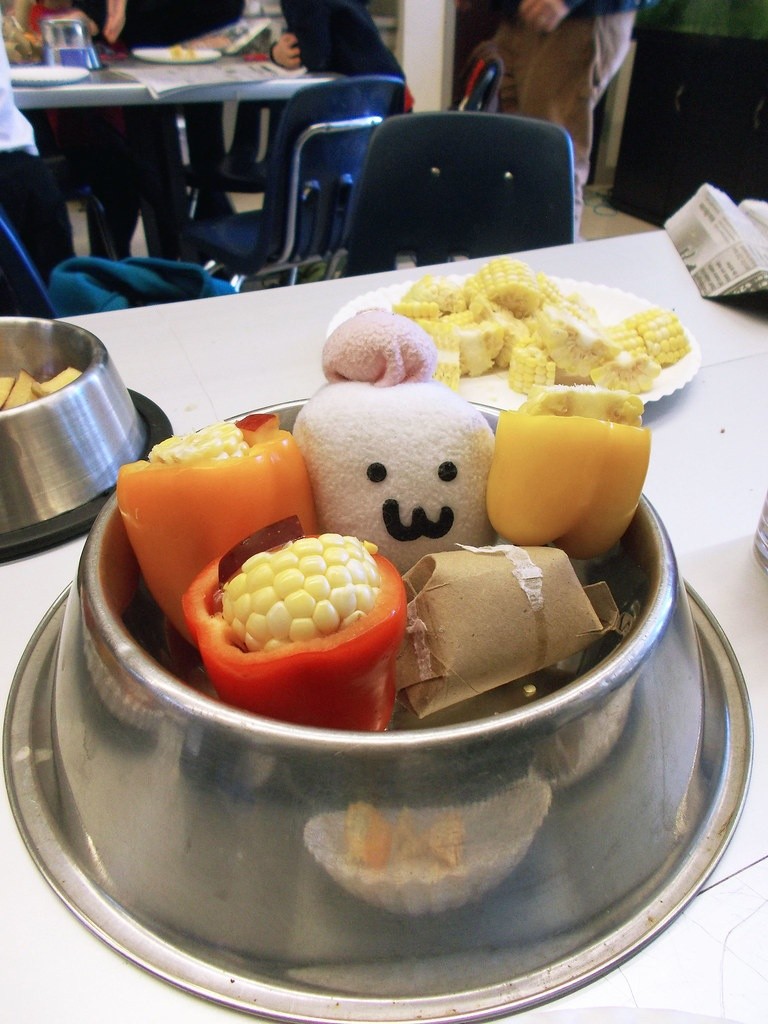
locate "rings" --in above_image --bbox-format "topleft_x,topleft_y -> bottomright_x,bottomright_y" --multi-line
538,16 -> 546,23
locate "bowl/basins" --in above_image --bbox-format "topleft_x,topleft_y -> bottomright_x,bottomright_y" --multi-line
39,19 -> 102,69
0,312 -> 176,560
0,393 -> 754,1024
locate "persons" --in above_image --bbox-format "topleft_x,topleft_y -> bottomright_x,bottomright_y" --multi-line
269,0 -> 414,115
453,0 -> 654,232
0,0 -> 245,260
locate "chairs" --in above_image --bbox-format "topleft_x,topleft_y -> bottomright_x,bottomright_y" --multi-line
183,75 -> 404,295
0,206 -> 58,319
340,113 -> 573,278
450,44 -> 503,114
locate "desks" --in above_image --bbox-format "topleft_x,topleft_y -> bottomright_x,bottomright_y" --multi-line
7,59 -> 344,110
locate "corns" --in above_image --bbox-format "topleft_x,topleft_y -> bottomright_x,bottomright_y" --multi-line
393,258 -> 690,397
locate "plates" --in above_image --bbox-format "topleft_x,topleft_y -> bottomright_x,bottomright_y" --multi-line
328,276 -> 701,411
132,48 -> 222,64
10,66 -> 90,85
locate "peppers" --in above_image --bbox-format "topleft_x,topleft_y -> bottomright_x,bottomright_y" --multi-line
488,385 -> 650,556
184,536 -> 406,732
118,413 -> 312,648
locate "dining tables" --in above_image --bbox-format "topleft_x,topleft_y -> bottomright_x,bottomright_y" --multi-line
0,231 -> 768,1024
